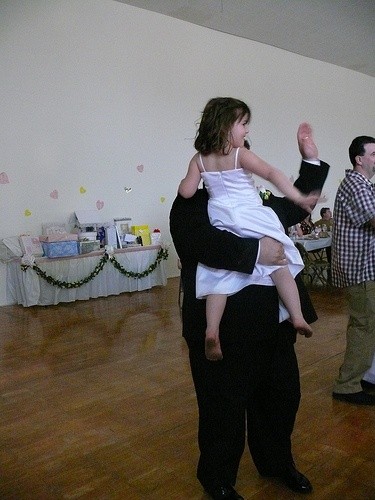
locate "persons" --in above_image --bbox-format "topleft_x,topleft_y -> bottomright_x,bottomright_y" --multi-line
169,122 -> 331,500
313,207 -> 334,281
179,96 -> 325,361
295,213 -> 315,236
331,136 -> 375,404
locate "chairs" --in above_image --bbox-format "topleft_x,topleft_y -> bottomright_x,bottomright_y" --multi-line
295,240 -> 331,287
312,249 -> 329,271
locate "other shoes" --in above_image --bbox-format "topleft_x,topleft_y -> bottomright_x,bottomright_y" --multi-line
332,391 -> 375,406
360,379 -> 375,391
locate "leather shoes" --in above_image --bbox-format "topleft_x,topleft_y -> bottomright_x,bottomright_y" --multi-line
270,467 -> 313,494
204,485 -> 244,500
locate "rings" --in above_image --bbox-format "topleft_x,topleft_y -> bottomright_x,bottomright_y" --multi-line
303,136 -> 309,140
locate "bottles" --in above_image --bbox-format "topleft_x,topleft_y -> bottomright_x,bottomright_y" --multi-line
288,226 -> 298,240
318,225 -> 322,233
323,224 -> 327,233
315,227 -> 318,235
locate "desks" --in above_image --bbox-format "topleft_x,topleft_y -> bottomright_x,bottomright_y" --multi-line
288,232 -> 335,291
1,238 -> 167,307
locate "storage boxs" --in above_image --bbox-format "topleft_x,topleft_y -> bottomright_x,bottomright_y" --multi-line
3,208 -> 163,259
